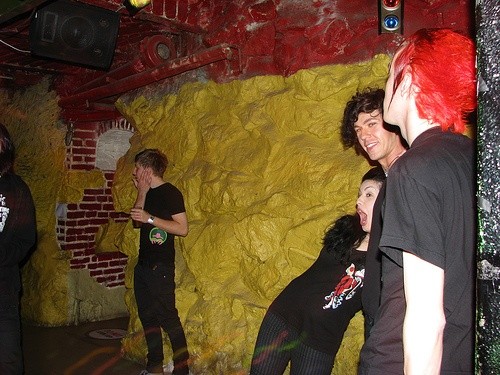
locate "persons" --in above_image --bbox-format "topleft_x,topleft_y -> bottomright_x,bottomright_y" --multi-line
130,148 -> 189,375
340,86 -> 408,342
355,25 -> 476,375
0,123 -> 37,375
250,166 -> 387,375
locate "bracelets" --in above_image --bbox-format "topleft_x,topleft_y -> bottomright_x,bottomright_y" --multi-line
148,215 -> 155,224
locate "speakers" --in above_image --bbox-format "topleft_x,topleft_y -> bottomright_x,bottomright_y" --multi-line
380,0 -> 402,35
27,1 -> 121,69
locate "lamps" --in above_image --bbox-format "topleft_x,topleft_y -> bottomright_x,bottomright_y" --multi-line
123,0 -> 152,17
